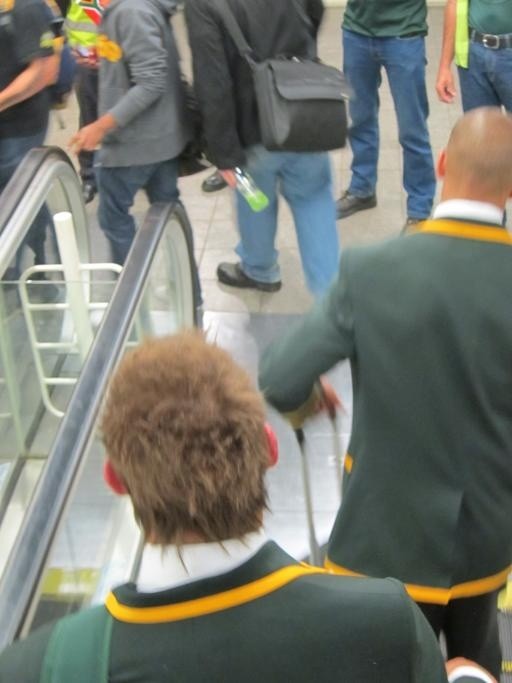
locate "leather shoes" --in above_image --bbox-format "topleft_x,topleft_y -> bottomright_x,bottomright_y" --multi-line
203,171 -> 228,192
334,190 -> 375,220
82,183 -> 97,203
400,218 -> 425,235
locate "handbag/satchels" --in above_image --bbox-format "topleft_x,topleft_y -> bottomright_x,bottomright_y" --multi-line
255,60 -> 354,152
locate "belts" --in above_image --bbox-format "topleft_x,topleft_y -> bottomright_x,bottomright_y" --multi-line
468,26 -> 511,50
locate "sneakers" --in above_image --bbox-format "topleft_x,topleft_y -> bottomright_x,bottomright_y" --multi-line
218,263 -> 280,290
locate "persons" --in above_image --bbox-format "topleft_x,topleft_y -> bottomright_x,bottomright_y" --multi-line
263,105 -> 508,682
436,0 -> 511,116
0,0 -> 190,276
1,321 -> 499,681
333,0 -> 436,240
181,0 -> 341,300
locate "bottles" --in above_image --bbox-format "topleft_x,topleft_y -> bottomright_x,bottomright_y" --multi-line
221,167 -> 274,213
